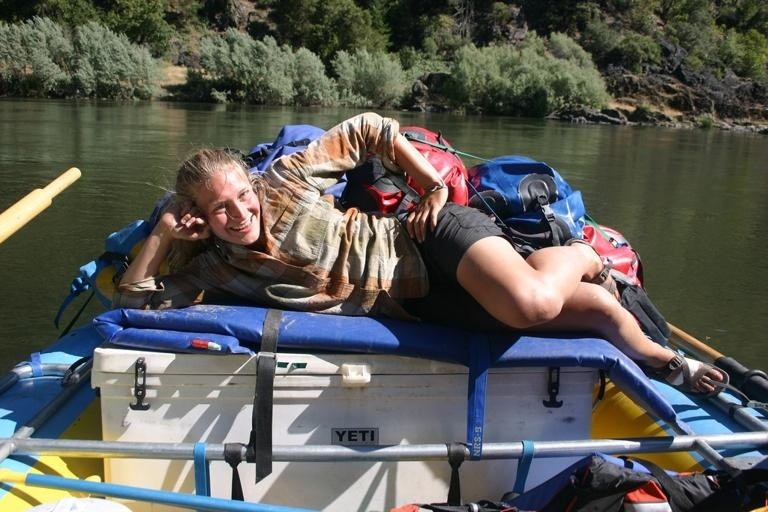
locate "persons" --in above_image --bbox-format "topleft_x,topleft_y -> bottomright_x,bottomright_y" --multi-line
111,109 -> 734,397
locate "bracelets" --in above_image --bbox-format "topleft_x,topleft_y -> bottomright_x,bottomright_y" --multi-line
422,184 -> 447,193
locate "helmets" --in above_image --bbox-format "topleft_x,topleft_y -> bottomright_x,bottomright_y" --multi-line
583,225 -> 642,288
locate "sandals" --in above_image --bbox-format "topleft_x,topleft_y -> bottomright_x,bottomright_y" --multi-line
647,354 -> 730,400
564,237 -> 622,304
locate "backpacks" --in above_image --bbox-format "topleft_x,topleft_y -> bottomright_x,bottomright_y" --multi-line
467,153 -> 581,255
56,126 -> 352,311
351,125 -> 470,218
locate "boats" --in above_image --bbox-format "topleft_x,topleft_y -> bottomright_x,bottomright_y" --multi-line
0,314 -> 768,512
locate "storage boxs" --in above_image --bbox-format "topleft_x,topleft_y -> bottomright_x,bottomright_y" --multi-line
90,346 -> 599,512
